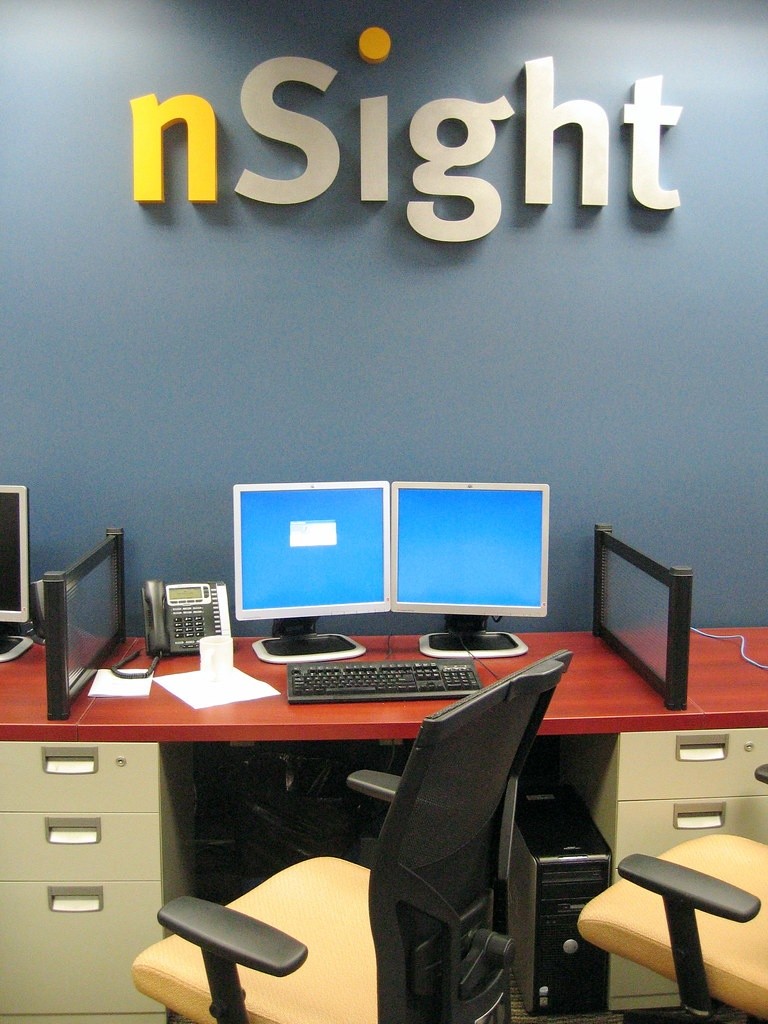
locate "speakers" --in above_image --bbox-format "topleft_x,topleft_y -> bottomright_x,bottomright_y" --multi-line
28,579 -> 46,645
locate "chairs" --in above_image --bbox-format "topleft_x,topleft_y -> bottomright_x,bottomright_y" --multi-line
133,648 -> 576,1024
578,765 -> 768,1024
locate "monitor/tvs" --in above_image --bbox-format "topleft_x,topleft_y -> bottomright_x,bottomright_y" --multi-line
0,484 -> 33,662
391,480 -> 550,658
232,479 -> 391,663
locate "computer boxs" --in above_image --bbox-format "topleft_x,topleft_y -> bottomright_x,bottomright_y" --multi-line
508,786 -> 611,1015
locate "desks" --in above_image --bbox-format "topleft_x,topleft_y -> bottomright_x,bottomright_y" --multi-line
619,626 -> 768,729
76,630 -> 707,743
0,635 -> 137,742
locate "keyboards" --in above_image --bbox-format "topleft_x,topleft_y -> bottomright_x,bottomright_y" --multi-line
286,658 -> 481,701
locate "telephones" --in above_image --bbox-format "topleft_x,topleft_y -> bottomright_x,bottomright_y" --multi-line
141,578 -> 232,656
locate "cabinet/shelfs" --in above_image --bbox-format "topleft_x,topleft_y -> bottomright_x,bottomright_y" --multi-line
0,740 -> 200,1024
556,727 -> 768,1024
